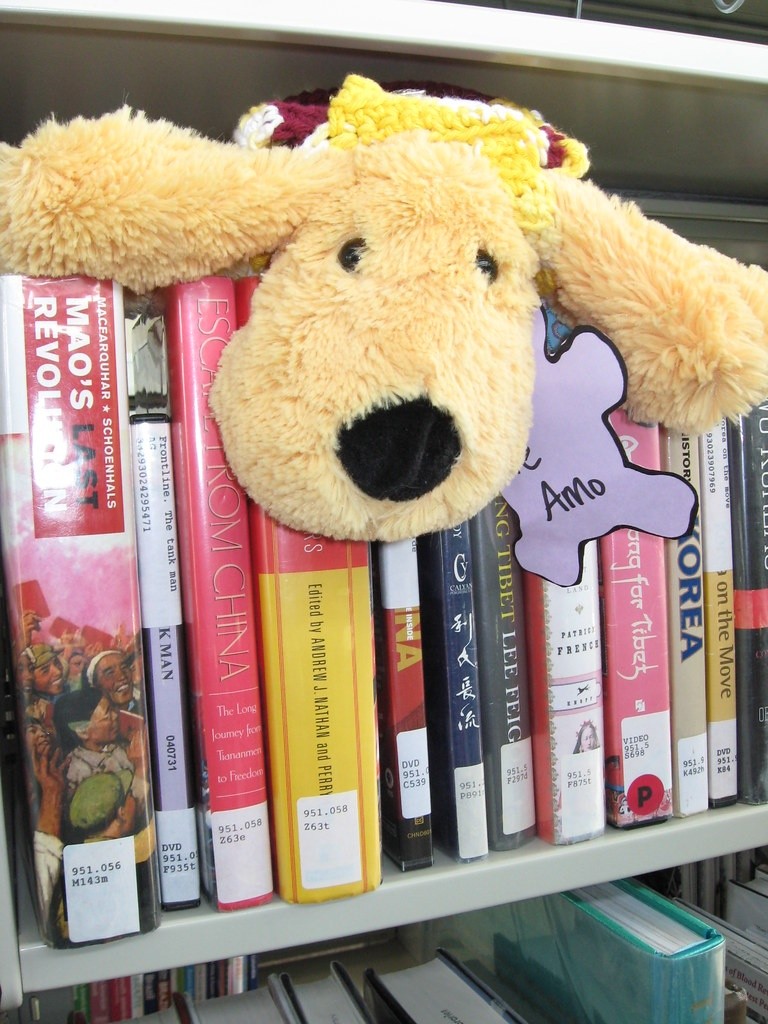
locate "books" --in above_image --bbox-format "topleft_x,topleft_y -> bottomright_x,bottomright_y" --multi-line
0,269 -> 768,1024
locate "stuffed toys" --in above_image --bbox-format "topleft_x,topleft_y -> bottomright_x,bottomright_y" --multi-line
0,72 -> 768,542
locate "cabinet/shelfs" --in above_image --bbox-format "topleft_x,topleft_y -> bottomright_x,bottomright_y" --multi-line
0,0 -> 767,1024
